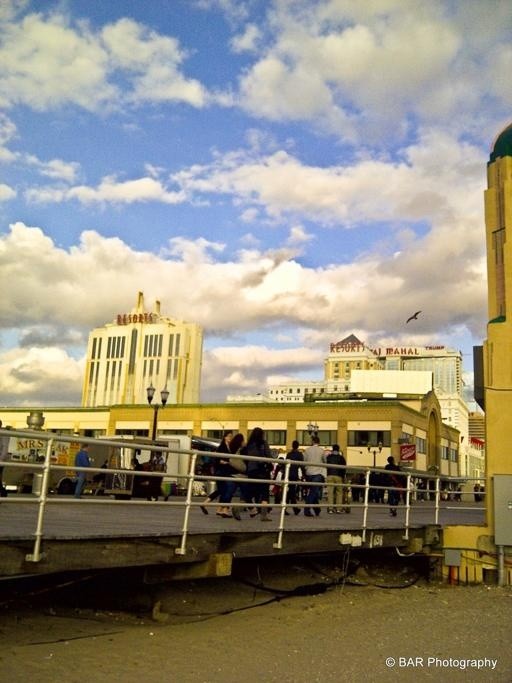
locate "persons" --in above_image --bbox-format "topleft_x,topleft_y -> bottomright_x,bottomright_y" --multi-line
74,443 -> 90,499
0,420 -> 10,497
130,456 -> 146,492
199,426 -> 486,522
101,459 -> 107,478
147,449 -> 170,502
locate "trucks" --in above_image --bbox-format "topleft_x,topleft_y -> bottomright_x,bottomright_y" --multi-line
0,421 -> 234,497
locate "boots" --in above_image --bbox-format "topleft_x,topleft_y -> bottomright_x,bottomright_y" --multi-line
200,497 -> 273,522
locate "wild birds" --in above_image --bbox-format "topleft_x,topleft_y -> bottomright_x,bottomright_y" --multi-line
406,311 -> 421,324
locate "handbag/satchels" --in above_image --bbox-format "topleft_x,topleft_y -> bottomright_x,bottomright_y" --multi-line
229,447 -> 246,473
263,461 -> 274,472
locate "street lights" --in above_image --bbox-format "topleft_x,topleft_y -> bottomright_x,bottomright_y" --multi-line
306,418 -> 320,447
368,439 -> 382,469
144,377 -> 170,463
